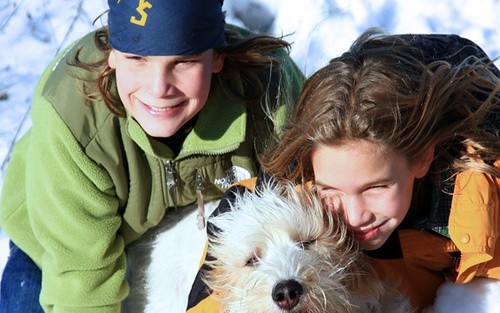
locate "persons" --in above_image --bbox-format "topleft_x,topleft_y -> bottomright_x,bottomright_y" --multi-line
185,26 -> 500,313
0,0 -> 308,313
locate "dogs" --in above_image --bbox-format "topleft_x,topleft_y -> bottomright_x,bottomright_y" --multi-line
200,172 -> 412,313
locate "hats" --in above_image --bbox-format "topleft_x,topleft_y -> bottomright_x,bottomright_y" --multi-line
108,0 -> 225,56
